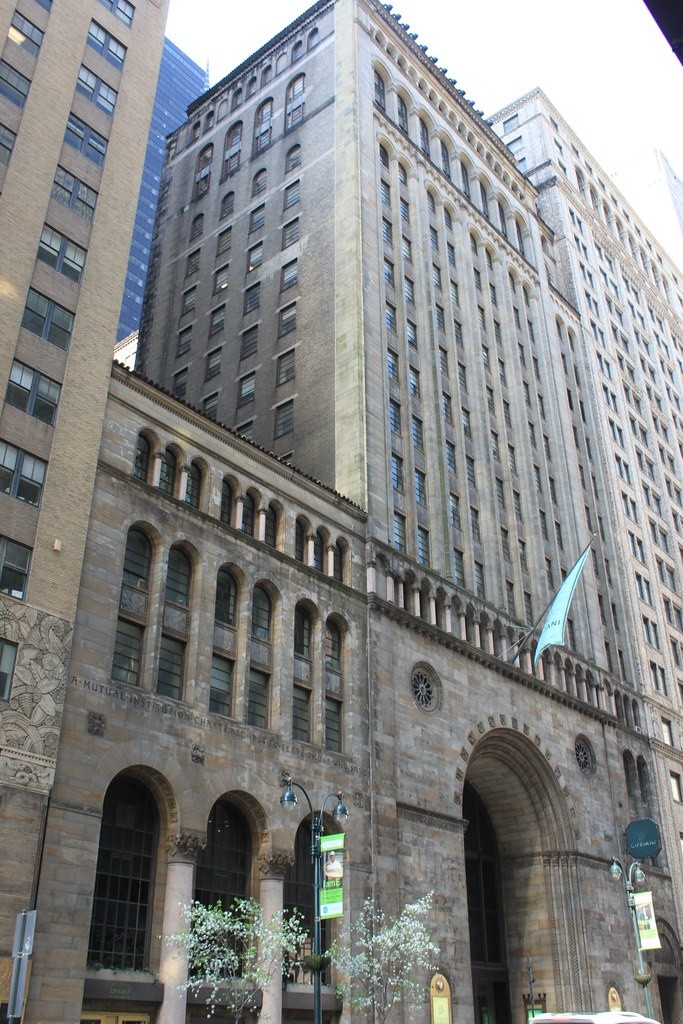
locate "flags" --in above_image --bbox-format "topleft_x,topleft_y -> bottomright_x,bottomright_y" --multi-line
534,545 -> 591,663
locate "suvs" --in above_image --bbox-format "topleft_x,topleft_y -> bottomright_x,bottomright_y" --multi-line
528,1012 -> 661,1024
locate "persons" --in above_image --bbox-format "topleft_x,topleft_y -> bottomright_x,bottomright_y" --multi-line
323,851 -> 340,881
640,906 -> 650,929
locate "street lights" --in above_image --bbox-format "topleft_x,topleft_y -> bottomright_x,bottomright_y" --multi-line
610,856 -> 655,1019
280,772 -> 350,1024
